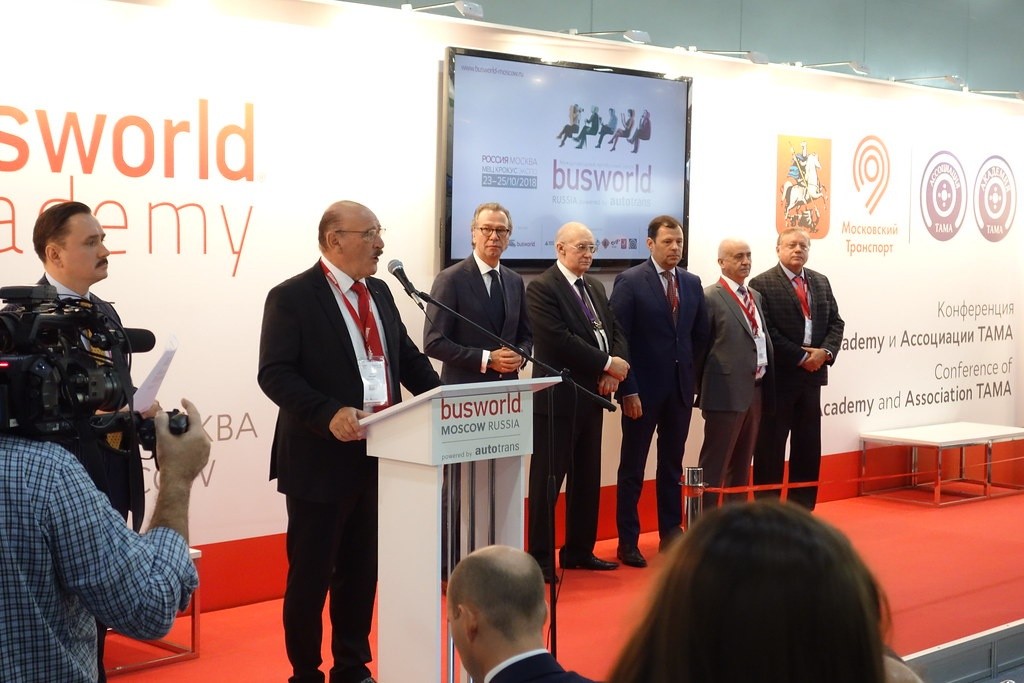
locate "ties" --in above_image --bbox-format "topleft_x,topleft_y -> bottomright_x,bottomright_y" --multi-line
575,277 -> 600,325
738,285 -> 763,372
661,270 -> 679,332
488,270 -> 506,334
794,277 -> 810,319
351,281 -> 392,413
78,300 -> 123,450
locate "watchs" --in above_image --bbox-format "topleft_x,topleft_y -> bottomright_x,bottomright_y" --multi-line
487,355 -> 492,365
825,350 -> 832,360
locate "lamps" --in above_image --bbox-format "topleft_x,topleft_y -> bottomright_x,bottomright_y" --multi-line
400,1 -> 483,18
689,45 -> 769,65
971,90 -> 1024,101
789,61 -> 869,75
558,28 -> 651,44
887,75 -> 969,92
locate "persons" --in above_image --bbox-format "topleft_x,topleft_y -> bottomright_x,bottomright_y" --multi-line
423,203 -> 533,386
607,499 -> 926,683
696,237 -> 777,517
608,214 -> 706,567
445,544 -> 605,683
256,202 -> 446,683
0,203 -> 145,683
0,337 -> 210,683
527,221 -> 631,585
749,229 -> 846,514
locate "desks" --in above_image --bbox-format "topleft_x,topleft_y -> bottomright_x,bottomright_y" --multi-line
858,422 -> 1024,508
105,548 -> 202,674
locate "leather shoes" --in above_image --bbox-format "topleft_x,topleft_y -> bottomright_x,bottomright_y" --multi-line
618,540 -> 647,568
542,567 -> 560,585
560,553 -> 621,571
658,530 -> 684,554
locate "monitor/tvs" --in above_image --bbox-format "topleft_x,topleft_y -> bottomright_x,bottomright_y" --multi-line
438,46 -> 694,274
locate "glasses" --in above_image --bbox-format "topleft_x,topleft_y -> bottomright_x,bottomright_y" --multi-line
475,227 -> 512,239
335,227 -> 387,243
562,240 -> 597,254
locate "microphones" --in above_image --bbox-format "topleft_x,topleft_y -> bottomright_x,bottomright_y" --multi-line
388,259 -> 424,309
89,326 -> 156,353
